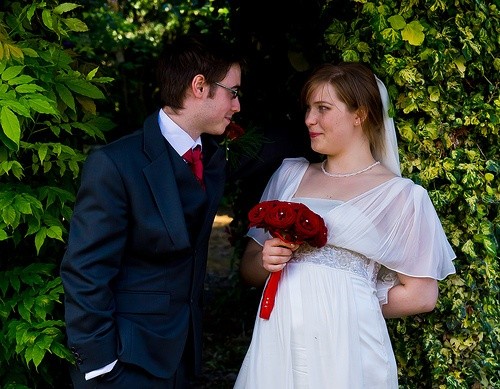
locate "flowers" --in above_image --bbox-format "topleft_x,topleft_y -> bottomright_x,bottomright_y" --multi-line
219,123 -> 274,168
247,200 -> 327,320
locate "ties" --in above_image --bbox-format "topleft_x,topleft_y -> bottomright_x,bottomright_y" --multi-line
182,148 -> 203,182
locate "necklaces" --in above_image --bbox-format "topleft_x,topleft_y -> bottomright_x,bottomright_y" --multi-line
320,157 -> 380,178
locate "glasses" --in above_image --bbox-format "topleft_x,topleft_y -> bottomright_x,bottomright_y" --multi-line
215,82 -> 240,99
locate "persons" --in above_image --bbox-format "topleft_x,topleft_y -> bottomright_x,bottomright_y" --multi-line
233,64 -> 456,389
59,42 -> 276,389
